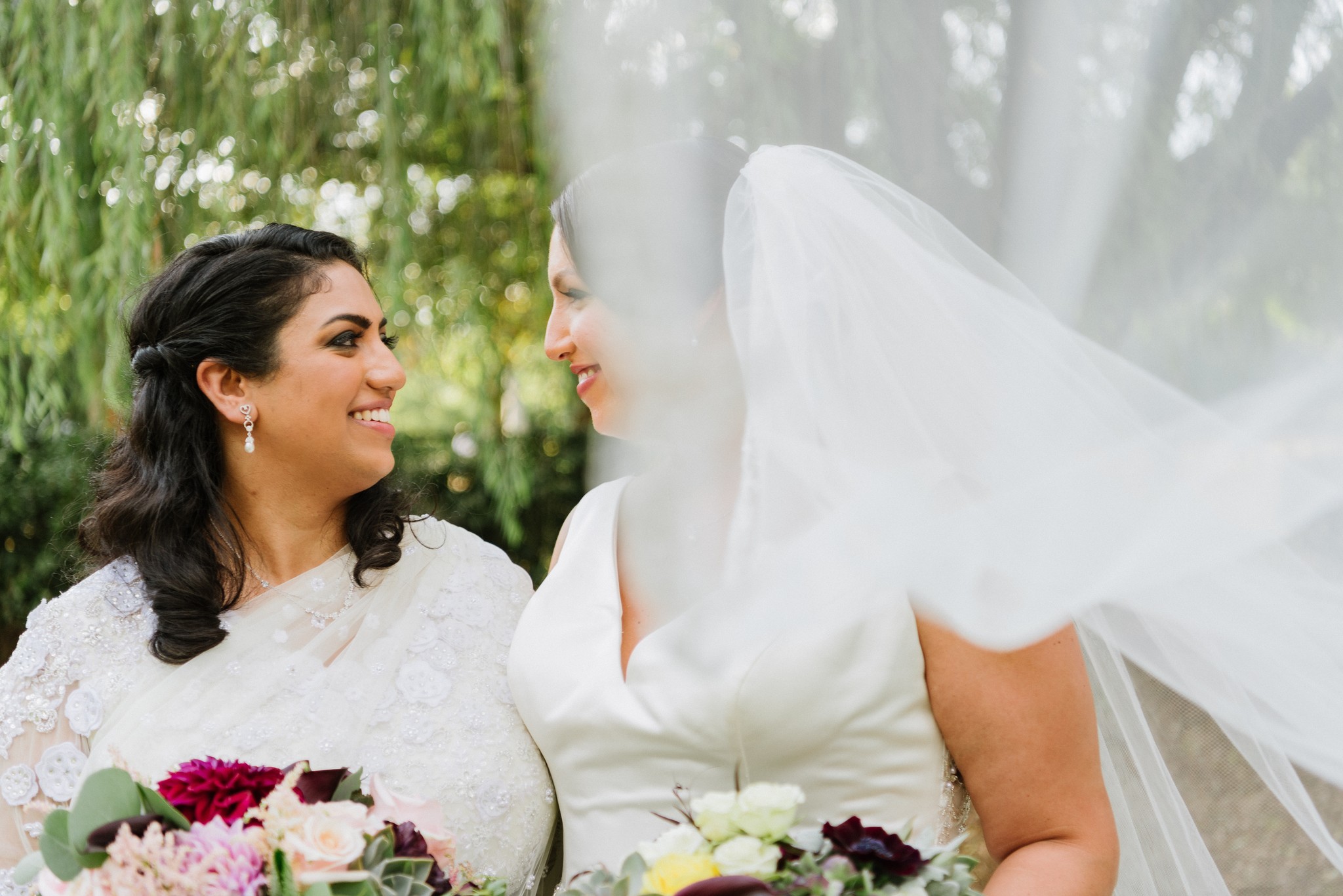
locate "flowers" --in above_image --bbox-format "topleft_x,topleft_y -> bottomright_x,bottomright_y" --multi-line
31,757 -> 496,894
548,786 -> 977,895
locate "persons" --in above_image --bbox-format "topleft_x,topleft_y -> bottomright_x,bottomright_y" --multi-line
0,214 -> 558,896
502,131 -> 1124,895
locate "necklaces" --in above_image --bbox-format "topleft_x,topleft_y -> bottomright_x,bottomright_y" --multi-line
204,511 -> 358,630
